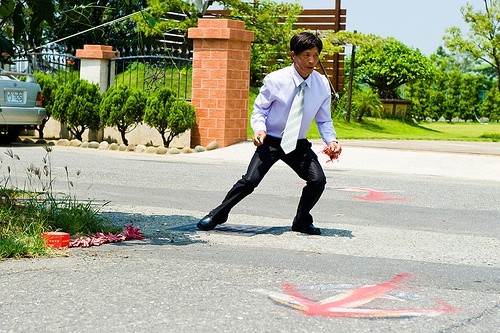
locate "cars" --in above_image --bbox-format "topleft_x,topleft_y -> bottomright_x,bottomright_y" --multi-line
0,65 -> 47,145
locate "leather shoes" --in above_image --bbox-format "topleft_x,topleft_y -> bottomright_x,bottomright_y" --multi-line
197,208 -> 228,231
291,218 -> 321,235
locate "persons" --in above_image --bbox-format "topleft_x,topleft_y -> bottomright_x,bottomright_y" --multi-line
197,32 -> 342,235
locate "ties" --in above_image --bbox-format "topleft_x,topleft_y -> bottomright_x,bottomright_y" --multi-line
279,82 -> 308,154
